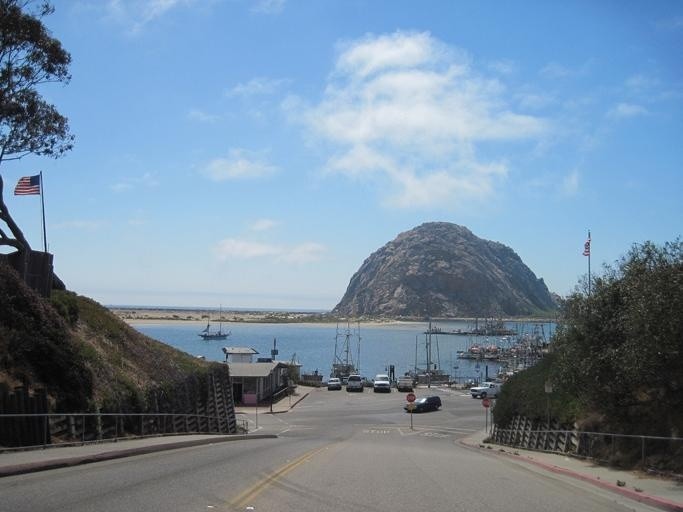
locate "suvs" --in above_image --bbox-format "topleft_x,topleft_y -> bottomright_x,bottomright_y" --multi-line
373,374 -> 390,392
404,395 -> 441,412
346,375 -> 363,391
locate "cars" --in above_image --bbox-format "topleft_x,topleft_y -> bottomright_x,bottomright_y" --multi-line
328,378 -> 341,390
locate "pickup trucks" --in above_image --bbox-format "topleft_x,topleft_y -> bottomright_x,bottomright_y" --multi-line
397,375 -> 413,391
470,382 -> 501,399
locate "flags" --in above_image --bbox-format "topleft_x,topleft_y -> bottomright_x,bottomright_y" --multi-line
583,238 -> 590,256
13,174 -> 41,196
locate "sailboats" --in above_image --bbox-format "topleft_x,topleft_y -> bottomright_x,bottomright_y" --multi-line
405,316 -> 451,384
197,303 -> 231,338
331,317 -> 365,384
456,321 -> 551,385
424,313 -> 520,335
